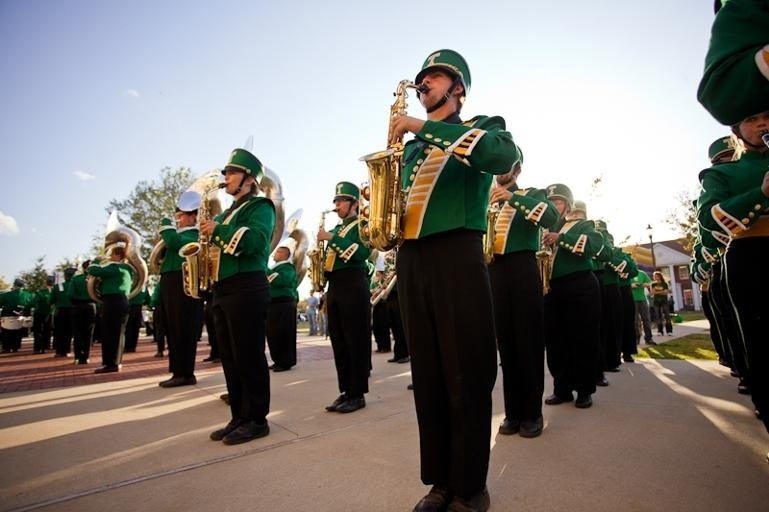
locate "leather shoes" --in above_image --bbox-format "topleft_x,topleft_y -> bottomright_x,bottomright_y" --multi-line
375,348 -> 410,363
545,392 -> 574,405
95,363 -> 118,373
1,348 -> 17,353
719,355 -> 764,420
269,363 -> 293,371
160,373 -> 196,387
211,417 -> 270,445
498,416 -> 543,437
204,356 -> 221,363
596,354 -> 634,386
576,392 -> 592,408
33,349 -> 87,363
412,483 -> 490,511
326,395 -> 366,412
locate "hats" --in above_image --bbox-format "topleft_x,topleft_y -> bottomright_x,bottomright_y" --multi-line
414,50 -> 471,100
14,278 -> 26,289
46,268 -> 78,283
547,184 -> 608,228
709,136 -> 744,163
333,181 -> 360,204
220,149 -> 263,179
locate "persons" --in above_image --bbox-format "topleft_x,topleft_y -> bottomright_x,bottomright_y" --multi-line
306,180 -> 410,413
0,246 -> 159,375
688,0 -> 768,456
151,206 -> 221,389
198,148 -> 277,444
367,47 -> 521,512
482,146 -> 672,438
263,247 -> 297,373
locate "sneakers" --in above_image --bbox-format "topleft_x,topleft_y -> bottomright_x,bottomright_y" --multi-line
657,332 -> 673,336
155,352 -> 163,357
646,339 -> 656,345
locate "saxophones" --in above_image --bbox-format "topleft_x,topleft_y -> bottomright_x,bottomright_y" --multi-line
178,181 -> 229,299
307,208 -> 337,294
536,225 -> 551,296
358,77 -> 429,253
482,179 -> 500,264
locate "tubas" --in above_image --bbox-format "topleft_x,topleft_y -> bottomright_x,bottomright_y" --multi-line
149,169 -> 222,276
259,165 -> 285,256
87,208 -> 148,303
283,207 -> 307,288
